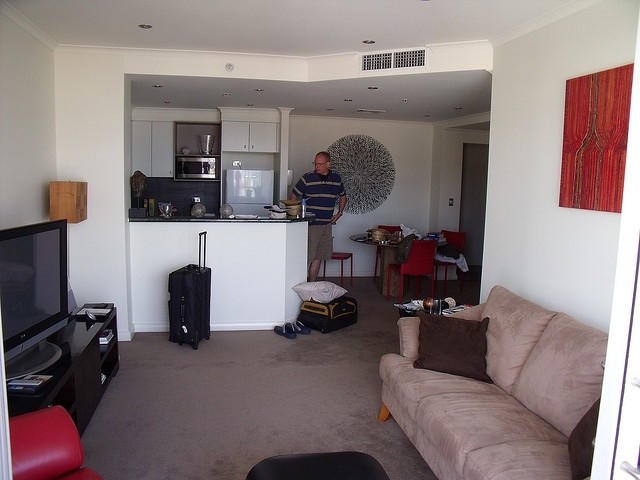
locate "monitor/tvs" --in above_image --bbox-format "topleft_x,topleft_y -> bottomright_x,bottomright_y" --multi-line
0,218 -> 68,381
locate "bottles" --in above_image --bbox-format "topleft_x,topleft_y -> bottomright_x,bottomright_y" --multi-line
143,197 -> 149,217
148,199 -> 155,217
368,227 -> 372,241
301,199 -> 306,218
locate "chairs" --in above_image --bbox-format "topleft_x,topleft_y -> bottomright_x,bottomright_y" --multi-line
405,229 -> 467,299
387,239 -> 437,304
373,224 -> 402,283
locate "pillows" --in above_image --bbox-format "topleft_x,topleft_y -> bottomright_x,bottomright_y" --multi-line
413,309 -> 495,384
291,281 -> 349,304
567,397 -> 600,480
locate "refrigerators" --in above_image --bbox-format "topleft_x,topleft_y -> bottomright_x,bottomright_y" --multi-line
224,170 -> 274,217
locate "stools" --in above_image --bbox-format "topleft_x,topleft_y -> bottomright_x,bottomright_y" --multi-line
323,252 -> 353,288
246,451 -> 389,480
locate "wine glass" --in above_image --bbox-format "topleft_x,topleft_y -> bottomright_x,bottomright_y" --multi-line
163,205 -> 171,218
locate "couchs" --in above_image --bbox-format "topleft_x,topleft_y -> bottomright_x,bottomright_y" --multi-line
377,284 -> 609,480
9,405 -> 108,480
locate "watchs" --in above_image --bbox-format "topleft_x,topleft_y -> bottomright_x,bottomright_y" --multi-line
338,211 -> 343,217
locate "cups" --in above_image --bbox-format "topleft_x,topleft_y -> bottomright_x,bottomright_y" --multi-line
158,202 -> 171,216
430,299 -> 442,316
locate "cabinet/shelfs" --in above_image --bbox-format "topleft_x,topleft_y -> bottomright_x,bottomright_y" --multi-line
225,169 -> 275,205
5,303 -> 120,439
173,120 -> 221,182
131,120 -> 176,178
222,120 -> 281,153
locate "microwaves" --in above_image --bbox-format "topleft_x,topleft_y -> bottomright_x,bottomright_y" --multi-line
177,158 -> 216,179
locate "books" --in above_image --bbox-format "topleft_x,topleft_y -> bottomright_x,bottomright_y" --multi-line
425,232 -> 446,243
99,329 -> 114,345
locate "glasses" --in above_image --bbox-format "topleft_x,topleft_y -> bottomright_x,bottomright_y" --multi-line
311,162 -> 328,166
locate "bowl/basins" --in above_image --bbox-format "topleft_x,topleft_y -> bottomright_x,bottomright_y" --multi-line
269,209 -> 286,219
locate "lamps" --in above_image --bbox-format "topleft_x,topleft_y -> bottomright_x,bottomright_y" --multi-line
287,169 -> 292,186
49,180 -> 88,279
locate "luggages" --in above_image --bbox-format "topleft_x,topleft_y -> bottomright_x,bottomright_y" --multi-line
168,231 -> 211,351
296,296 -> 358,334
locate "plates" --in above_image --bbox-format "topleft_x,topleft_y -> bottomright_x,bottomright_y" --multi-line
279,200 -> 301,205
235,215 -> 258,219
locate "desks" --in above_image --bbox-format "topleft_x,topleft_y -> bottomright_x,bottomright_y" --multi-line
398,307 -> 416,316
393,297 -> 478,317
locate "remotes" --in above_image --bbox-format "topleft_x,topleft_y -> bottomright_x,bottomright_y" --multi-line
6,385 -> 37,395
7,380 -> 43,387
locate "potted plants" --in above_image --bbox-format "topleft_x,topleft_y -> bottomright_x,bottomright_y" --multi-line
128,170 -> 152,218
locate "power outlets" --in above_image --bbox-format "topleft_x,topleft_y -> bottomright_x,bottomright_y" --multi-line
232,160 -> 239,166
192,196 -> 201,203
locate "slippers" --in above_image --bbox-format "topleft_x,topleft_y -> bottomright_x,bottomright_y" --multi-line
293,321 -> 311,334
274,322 -> 296,339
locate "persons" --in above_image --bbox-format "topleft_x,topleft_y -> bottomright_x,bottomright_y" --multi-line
290,150 -> 347,282
